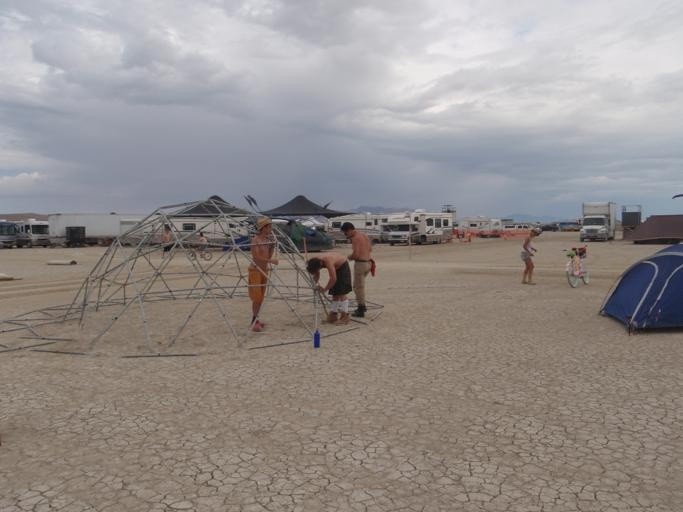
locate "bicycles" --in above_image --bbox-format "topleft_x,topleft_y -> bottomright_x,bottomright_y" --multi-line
563,245 -> 589,288
187,246 -> 212,261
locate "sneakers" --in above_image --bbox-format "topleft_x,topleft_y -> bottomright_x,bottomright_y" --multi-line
250,319 -> 264,331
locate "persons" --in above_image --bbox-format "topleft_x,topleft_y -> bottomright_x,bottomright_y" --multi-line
191,233 -> 208,259
161,226 -> 173,259
305,252 -> 352,324
519,229 -> 539,285
246,216 -> 279,331
340,222 -> 372,318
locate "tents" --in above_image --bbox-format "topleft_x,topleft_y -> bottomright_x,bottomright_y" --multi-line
598,244 -> 682,334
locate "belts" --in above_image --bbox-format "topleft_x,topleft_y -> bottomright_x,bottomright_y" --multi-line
358,260 -> 368,262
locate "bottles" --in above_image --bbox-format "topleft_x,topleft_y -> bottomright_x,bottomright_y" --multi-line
312,328 -> 321,348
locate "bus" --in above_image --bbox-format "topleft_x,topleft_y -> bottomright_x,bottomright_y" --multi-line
0,221 -> 16,248
502,224 -> 541,236
15,220 -> 49,248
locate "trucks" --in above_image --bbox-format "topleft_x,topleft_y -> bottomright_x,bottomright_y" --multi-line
578,202 -> 615,242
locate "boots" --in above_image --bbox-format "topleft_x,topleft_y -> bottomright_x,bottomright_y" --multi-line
322,311 -> 351,325
351,303 -> 366,317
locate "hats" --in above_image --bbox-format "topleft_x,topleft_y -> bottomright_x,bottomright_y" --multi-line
256,217 -> 272,231
